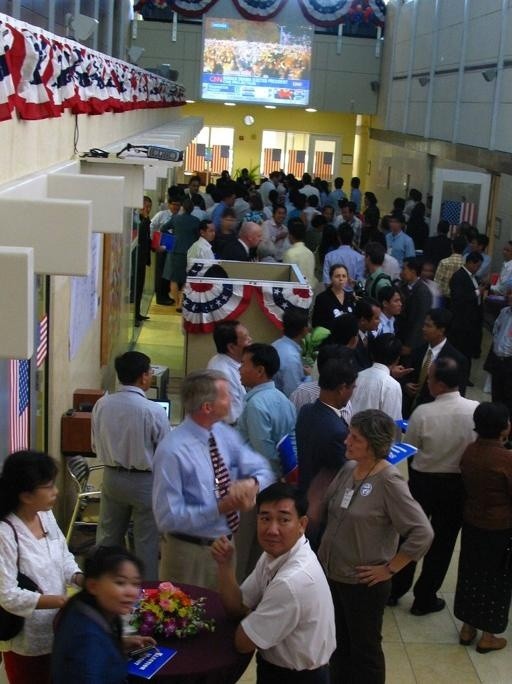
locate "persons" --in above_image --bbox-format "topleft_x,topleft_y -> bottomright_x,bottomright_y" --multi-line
184,176 -> 206,204
353,296 -> 381,365
347,334 -> 403,427
386,361 -> 481,616
150,369 -> 275,593
399,309 -> 472,421
350,175 -> 363,215
361,243 -> 392,304
484,287 -> 512,422
359,207 -> 387,256
243,195 -> 266,225
150,194 -> 180,306
258,170 -> 283,209
490,241 -> 512,297
282,224 -> 316,293
129,197 -> 164,328
363,191 -> 380,225
454,402 -> 512,653
206,320 -> 253,428
398,255 -> 435,418
368,230 -> 401,284
263,190 -> 281,218
297,172 -> 321,206
271,306 -> 309,399
336,203 -> 364,254
312,264 -> 357,348
287,194 -> 309,225
191,194 -> 208,220
432,237 -> 467,313
208,482 -> 337,684
236,168 -> 253,188
374,285 -> 403,337
204,180 -> 216,199
51,546 -> 142,682
220,222 -> 263,262
308,409 -> 435,684
322,205 -> 337,226
461,233 -> 492,280
381,186 -> 422,227
406,202 -> 431,250
210,210 -> 236,261
310,214 -> 341,267
325,174 -> 347,207
290,344 -> 358,412
89,352 -> 170,582
0,450 -> 83,683
316,178 -> 329,206
302,195 -> 321,222
285,178 -> 300,202
296,361 -> 358,518
422,221 -> 452,270
205,190 -> 224,220
161,199 -> 200,312
419,261 -> 443,311
448,250 -> 483,388
323,222 -> 366,290
234,343 -> 299,482
259,205 -> 292,261
187,219 -> 216,260
214,192 -> 237,228
232,191 -> 253,218
384,213 -> 417,271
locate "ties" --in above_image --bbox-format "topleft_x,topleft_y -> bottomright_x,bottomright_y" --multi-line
418,349 -> 433,388
207,432 -> 242,534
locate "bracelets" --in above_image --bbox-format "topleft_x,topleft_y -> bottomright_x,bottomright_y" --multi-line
383,561 -> 399,579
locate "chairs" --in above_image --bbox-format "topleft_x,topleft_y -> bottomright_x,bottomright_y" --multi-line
66,456 -> 130,551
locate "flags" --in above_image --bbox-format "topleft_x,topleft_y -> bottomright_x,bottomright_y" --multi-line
36,316 -> 49,369
7,356 -> 31,455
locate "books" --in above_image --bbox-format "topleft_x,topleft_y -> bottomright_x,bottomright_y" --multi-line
124,646 -> 177,679
385,442 -> 418,466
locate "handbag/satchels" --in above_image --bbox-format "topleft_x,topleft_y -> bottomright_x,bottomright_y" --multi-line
0,517 -> 45,642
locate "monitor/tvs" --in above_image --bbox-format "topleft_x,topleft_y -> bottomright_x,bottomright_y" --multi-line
147,399 -> 171,422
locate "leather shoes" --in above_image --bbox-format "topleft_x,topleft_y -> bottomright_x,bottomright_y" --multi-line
135,321 -> 140,327
138,314 -> 150,320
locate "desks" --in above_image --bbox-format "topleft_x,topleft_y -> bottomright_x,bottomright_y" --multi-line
53,581 -> 255,684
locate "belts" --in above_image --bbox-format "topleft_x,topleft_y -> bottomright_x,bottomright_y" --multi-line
169,531 -> 232,547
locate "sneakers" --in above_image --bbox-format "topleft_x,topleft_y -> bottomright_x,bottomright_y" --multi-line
475,636 -> 508,655
156,298 -> 175,305
459,628 -> 479,646
409,597 -> 447,616
